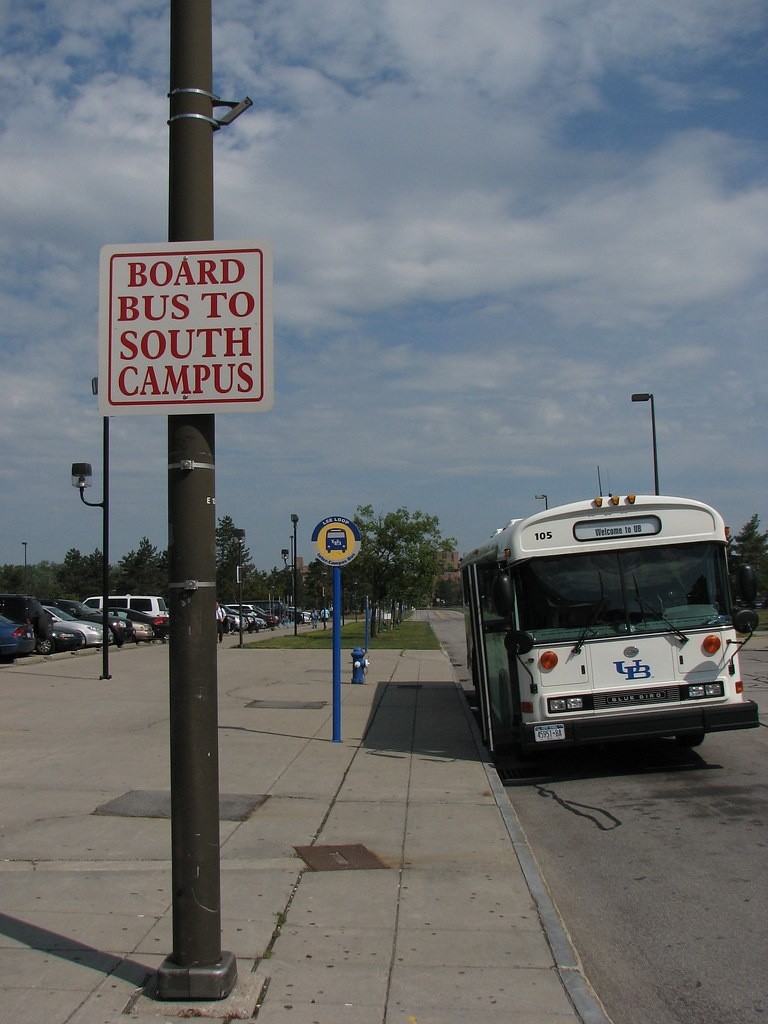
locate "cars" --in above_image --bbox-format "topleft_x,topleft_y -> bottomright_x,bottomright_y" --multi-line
214,600 -> 322,631
36,599 -> 170,655
0,614 -> 36,656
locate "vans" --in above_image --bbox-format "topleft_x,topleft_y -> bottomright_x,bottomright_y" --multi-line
82,594 -> 168,618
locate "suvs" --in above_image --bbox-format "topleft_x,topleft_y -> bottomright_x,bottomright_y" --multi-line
0,594 -> 54,647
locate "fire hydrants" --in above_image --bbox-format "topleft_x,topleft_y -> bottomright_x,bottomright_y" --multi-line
350,646 -> 371,684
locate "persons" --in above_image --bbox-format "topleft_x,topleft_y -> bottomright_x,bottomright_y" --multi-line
309,608 -> 320,628
320,607 -> 330,629
215,602 -> 226,644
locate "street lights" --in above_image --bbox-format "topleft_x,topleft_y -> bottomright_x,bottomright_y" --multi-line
631,392 -> 659,496
70,376 -> 112,681
534,494 -> 548,510
281,513 -> 298,636
233,528 -> 246,648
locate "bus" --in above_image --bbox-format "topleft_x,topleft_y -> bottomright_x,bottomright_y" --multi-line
458,495 -> 761,754
